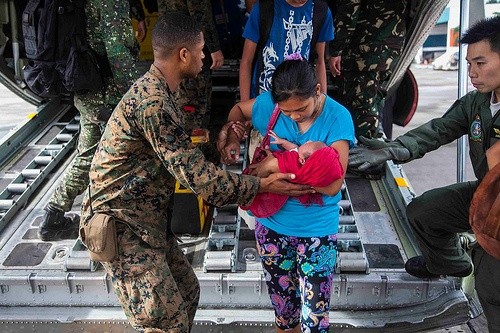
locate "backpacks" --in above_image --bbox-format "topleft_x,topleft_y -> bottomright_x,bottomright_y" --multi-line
20,0 -> 112,99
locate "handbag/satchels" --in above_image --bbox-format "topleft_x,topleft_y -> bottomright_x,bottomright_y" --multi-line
237,102 -> 282,210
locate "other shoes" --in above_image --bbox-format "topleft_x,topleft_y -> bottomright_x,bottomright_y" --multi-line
39,205 -> 79,241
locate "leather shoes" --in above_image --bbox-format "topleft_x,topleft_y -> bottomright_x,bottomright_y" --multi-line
405,255 -> 473,279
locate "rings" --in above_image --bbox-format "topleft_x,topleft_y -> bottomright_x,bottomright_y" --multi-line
224,157 -> 227,160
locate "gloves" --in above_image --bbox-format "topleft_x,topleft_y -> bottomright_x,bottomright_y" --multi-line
347,136 -> 410,171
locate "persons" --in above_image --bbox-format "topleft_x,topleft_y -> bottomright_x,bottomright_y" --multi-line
347,12 -> 500,333
36,0 -> 406,240
221,59 -> 355,333
83,10 -> 316,333
238,130 -> 343,218
